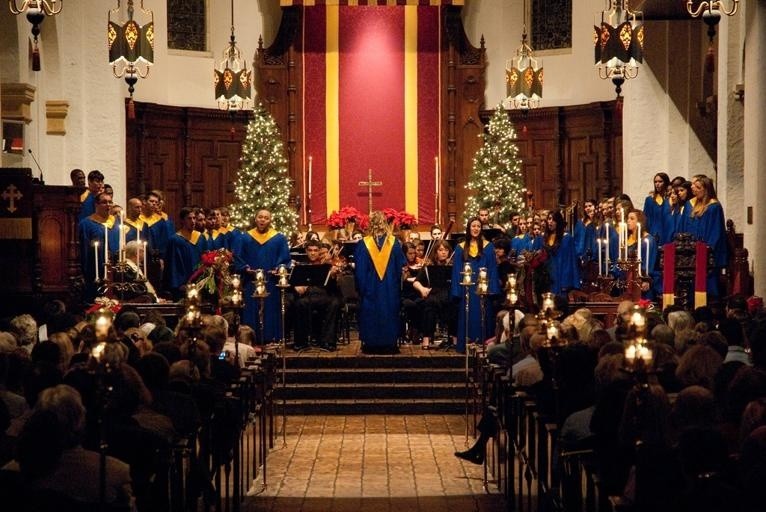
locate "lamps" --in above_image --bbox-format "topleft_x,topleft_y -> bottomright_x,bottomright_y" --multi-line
8,0 -> 62,72
460,262 -> 562,491
591,0 -> 649,110
505,0 -> 545,132
618,301 -> 654,493
109,0 -> 155,119
83,298 -> 130,489
686,0 -> 739,72
179,264 -> 290,457
214,0 -> 251,134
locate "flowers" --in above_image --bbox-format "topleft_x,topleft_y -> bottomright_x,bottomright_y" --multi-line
326,205 -> 417,230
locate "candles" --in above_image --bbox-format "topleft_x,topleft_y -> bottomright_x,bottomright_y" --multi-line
598,208 -> 649,278
94,211 -> 147,283
435,157 -> 439,193
308,156 -> 312,191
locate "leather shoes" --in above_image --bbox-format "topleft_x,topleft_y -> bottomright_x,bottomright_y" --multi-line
454,449 -> 484,465
292,336 -> 337,353
422,344 -> 429,349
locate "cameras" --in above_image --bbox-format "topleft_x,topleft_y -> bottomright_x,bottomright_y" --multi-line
219,351 -> 230,359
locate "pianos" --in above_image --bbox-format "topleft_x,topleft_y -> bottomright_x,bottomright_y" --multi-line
117,300 -> 245,321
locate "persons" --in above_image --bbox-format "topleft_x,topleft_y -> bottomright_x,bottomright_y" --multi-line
495,193 -> 659,300
163,204 -> 238,299
1,299 -> 260,512
286,231 -> 355,352
236,208 -> 292,344
354,210 -> 409,353
644,172 -> 727,296
70,170 -> 174,304
455,291 -> 766,510
478,207 -> 498,229
397,223 -> 457,349
451,217 -> 499,353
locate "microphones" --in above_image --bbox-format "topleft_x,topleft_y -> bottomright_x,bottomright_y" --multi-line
27,148 -> 43,182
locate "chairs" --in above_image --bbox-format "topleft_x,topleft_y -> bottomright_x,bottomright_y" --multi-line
333,292 -> 450,351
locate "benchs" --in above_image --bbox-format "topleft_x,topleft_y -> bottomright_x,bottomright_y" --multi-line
467,343 -> 633,511
0,350 -> 280,512
